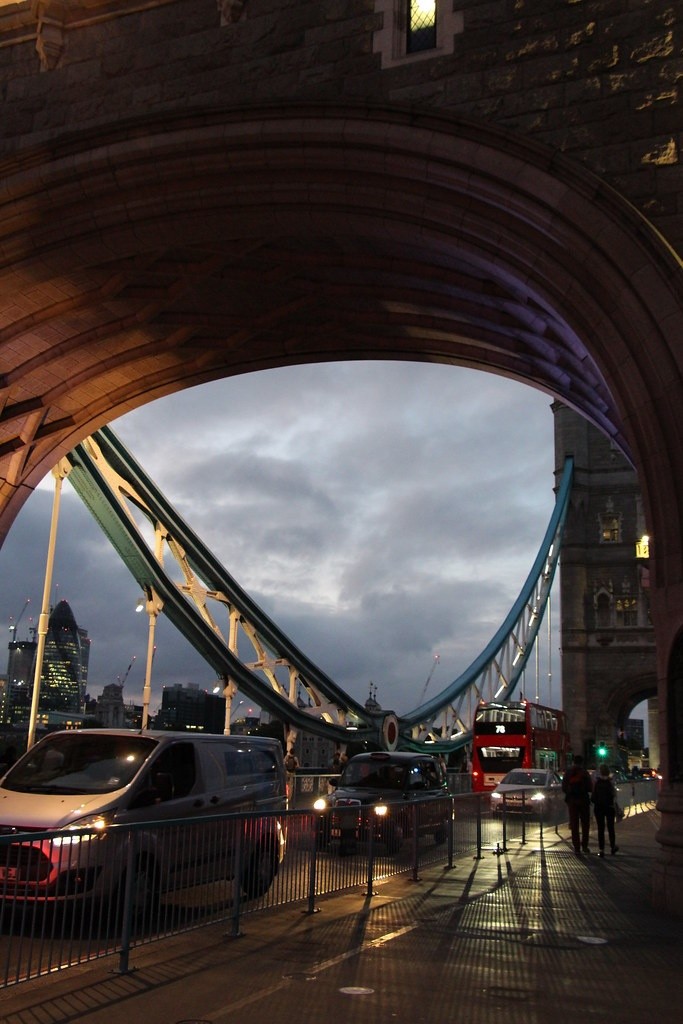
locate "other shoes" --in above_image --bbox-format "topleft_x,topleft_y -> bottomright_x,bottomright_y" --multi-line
574,850 -> 579,855
583,848 -> 589,852
598,852 -> 604,857
611,845 -> 618,853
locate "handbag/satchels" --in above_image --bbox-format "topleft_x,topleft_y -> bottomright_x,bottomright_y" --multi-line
613,801 -> 623,819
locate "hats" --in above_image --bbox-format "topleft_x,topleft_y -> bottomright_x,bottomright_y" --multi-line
600,764 -> 610,775
571,755 -> 584,762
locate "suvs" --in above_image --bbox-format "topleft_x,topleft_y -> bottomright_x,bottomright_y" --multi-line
314,751 -> 456,856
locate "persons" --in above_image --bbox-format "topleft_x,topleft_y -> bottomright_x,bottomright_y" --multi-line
438,757 -> 446,774
590,764 -> 619,858
284,748 -> 300,784
561,756 -> 594,855
327,753 -> 349,768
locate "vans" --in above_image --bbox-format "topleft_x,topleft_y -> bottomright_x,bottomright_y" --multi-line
2,728 -> 290,920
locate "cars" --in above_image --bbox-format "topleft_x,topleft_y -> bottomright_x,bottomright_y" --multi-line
491,769 -> 563,820
636,768 -> 657,781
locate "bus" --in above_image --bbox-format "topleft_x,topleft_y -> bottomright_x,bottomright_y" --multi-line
472,699 -> 571,795
